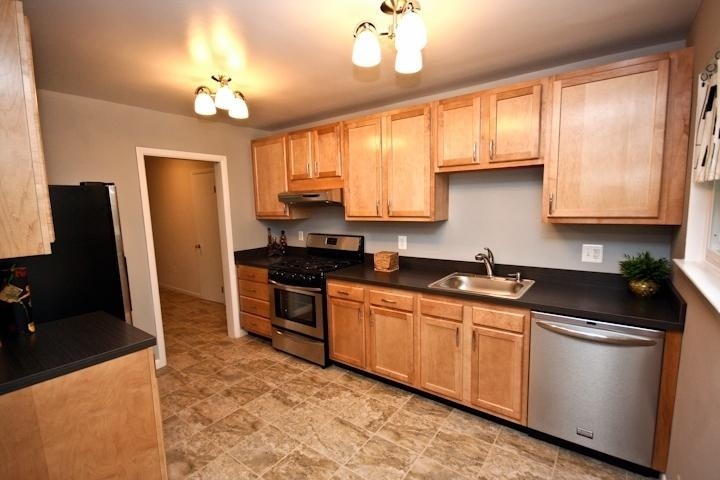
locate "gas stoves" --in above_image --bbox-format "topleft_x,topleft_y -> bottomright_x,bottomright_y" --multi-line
267,253 -> 353,287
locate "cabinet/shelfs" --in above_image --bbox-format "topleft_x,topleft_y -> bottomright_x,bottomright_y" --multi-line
0,313 -> 169,479
236,263 -> 273,340
541,44 -> 695,225
431,80 -> 544,174
324,277 -> 417,390
417,287 -> 530,428
251,130 -> 311,220
287,120 -> 343,192
343,101 -> 449,223
1,0 -> 55,261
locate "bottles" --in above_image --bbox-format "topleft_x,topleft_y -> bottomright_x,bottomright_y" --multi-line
271,237 -> 279,251
279,230 -> 288,256
267,227 -> 272,244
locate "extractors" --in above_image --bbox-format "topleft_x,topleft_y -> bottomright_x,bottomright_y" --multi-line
278,188 -> 343,208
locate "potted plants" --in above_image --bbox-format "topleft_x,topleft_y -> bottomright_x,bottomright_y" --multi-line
619,249 -> 674,298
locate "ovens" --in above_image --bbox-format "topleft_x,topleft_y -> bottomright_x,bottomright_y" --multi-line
266,278 -> 327,370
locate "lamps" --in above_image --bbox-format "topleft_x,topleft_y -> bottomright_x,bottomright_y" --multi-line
190,75 -> 254,121
351,0 -> 429,75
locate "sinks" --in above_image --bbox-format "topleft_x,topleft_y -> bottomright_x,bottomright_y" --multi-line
427,271 -> 536,300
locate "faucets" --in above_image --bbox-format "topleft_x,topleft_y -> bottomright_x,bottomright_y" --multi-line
475,247 -> 495,278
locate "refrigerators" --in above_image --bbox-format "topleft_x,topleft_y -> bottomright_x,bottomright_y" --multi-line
2,180 -> 135,330
